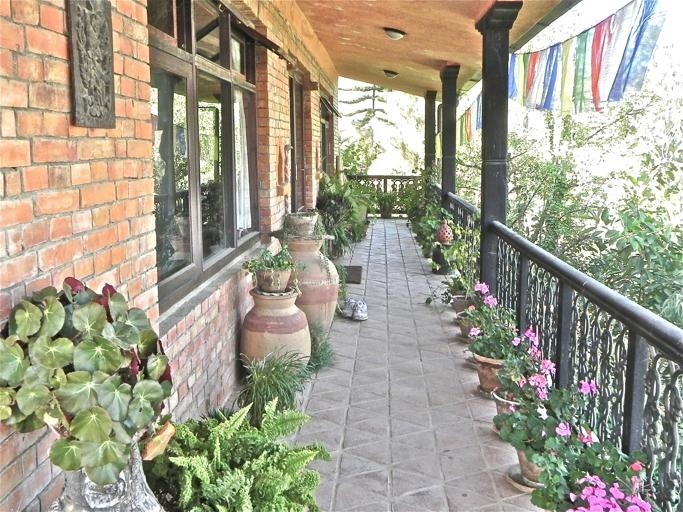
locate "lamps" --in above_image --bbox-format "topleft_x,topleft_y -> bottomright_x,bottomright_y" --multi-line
384,70 -> 399,80
383,27 -> 407,41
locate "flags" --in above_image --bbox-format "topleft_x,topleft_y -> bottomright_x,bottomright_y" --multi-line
458,1 -> 666,147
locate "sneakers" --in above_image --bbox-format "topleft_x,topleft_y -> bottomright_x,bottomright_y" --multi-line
340,296 -> 357,319
352,299 -> 369,321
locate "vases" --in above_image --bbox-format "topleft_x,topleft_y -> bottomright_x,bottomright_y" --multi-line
285,239 -> 340,357
473,353 -> 504,396
455,310 -> 476,343
240,289 -> 312,376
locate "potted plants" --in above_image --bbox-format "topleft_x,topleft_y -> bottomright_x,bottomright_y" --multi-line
283,202 -> 332,284
0,275 -> 173,512
242,242 -> 296,292
315,154 -> 480,312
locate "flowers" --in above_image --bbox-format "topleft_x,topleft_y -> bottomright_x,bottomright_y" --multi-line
494,324 -> 661,512
461,282 -> 522,360
